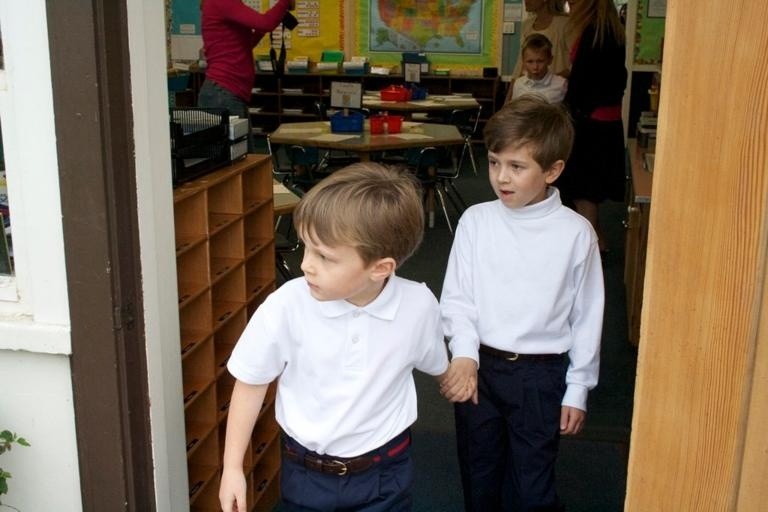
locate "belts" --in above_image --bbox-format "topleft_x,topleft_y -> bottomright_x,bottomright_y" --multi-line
282,443 -> 372,478
479,342 -> 570,363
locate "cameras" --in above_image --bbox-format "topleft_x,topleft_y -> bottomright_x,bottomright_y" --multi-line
282,12 -> 299,31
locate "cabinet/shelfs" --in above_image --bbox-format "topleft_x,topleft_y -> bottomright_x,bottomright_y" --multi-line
623,139 -> 652,347
173,153 -> 281,511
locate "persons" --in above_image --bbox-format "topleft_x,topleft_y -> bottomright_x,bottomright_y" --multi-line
565,2 -> 630,225
502,0 -> 574,102
197,1 -> 296,122
214,162 -> 476,512
509,31 -> 570,113
438,89 -> 608,512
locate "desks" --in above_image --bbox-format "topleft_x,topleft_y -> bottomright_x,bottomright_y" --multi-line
270,94 -> 480,280
